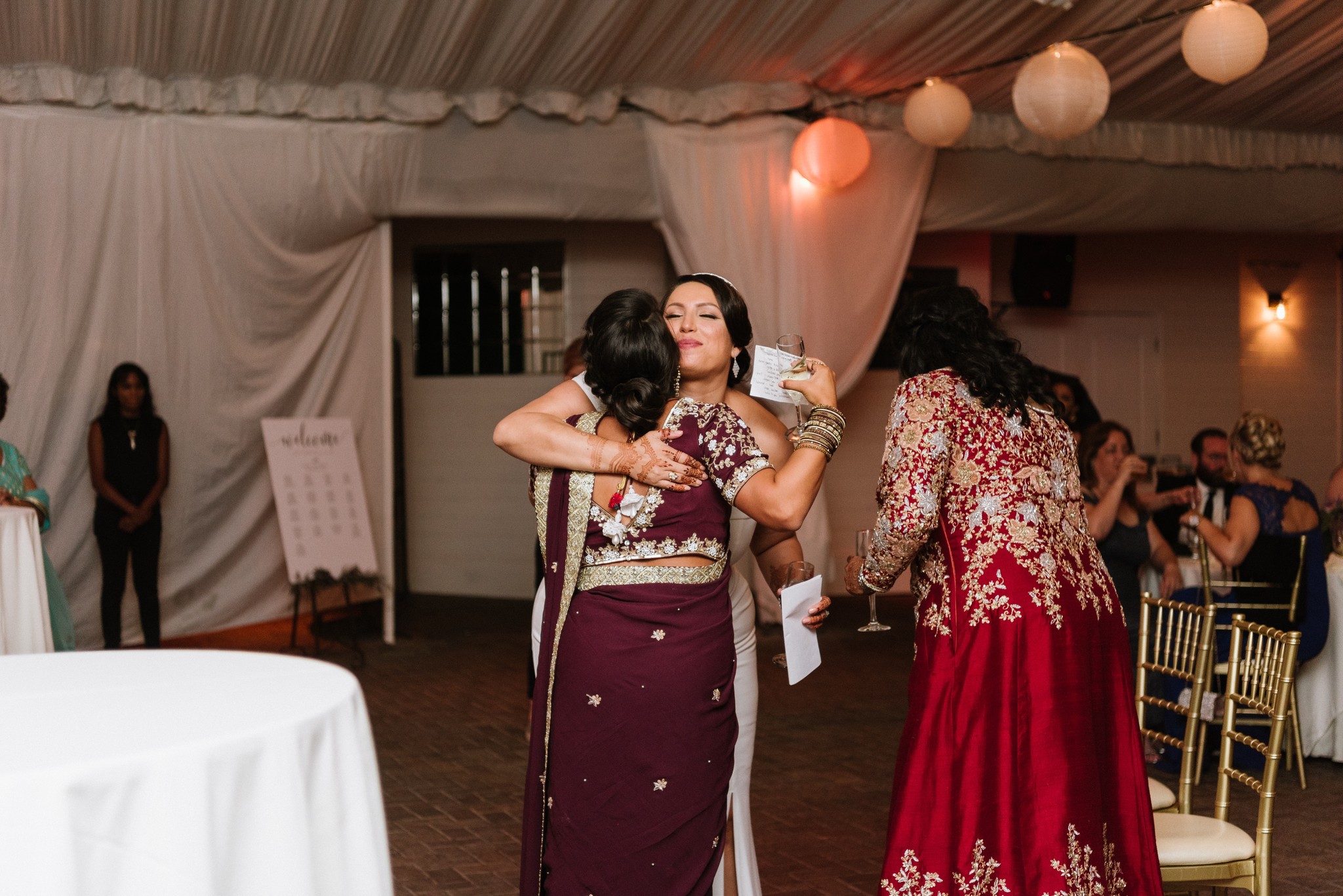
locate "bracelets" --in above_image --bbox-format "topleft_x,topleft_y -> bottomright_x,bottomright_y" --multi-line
794,404 -> 847,462
1188,514 -> 1204,530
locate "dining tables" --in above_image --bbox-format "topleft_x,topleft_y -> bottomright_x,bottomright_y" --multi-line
1139,557 -> 1235,604
1,650 -> 394,890
1277,554 -> 1343,767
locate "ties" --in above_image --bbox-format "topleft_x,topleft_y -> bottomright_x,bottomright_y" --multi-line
1204,486 -> 1218,522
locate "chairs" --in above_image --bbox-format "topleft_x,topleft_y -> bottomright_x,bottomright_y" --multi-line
1132,592 -> 1219,814
1193,530 -> 1310,790
1143,613 -> 1302,896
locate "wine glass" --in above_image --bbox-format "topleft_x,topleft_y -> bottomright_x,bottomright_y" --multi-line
771,559 -> 815,670
855,529 -> 891,632
774,334 -> 811,442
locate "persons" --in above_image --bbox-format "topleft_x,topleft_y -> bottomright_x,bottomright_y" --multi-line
492,273 -> 831,896
519,288 -> 848,896
87,362 -> 171,650
0,372 -> 79,651
1078,421 -> 1186,695
1152,410 -> 1330,776
1159,427 -> 1243,557
1325,463 -> 1343,505
845,285 -> 1162,896
1045,373 -> 1102,443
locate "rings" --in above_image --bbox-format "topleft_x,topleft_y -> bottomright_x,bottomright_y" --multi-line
669,473 -> 678,480
811,360 -> 824,371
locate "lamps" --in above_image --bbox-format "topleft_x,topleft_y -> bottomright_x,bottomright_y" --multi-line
1264,288 -> 1288,323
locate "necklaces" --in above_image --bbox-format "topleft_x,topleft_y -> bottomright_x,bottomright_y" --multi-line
127,429 -> 138,448
